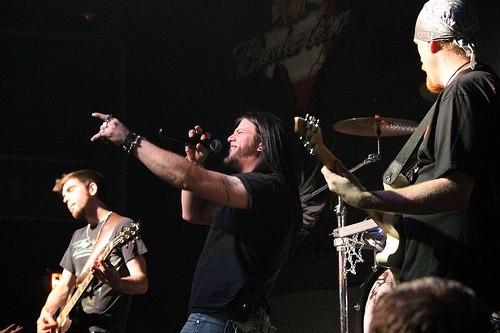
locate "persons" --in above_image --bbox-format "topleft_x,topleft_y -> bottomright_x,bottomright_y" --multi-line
37,170 -> 149,333
321,1 -> 500,333
91,112 -> 302,333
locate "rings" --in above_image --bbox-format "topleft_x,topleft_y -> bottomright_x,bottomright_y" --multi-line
102,116 -> 111,128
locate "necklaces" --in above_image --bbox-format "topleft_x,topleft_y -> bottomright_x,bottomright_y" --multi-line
446,62 -> 470,86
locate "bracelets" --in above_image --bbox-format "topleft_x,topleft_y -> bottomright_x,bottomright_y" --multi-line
122,133 -> 141,155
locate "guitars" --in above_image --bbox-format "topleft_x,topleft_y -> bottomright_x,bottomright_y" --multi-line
294,113 -> 405,269
52,220 -> 142,333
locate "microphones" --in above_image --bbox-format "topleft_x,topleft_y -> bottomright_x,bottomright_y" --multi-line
159,128 -> 222,153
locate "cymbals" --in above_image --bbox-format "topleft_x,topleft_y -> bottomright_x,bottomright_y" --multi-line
332,116 -> 419,137
